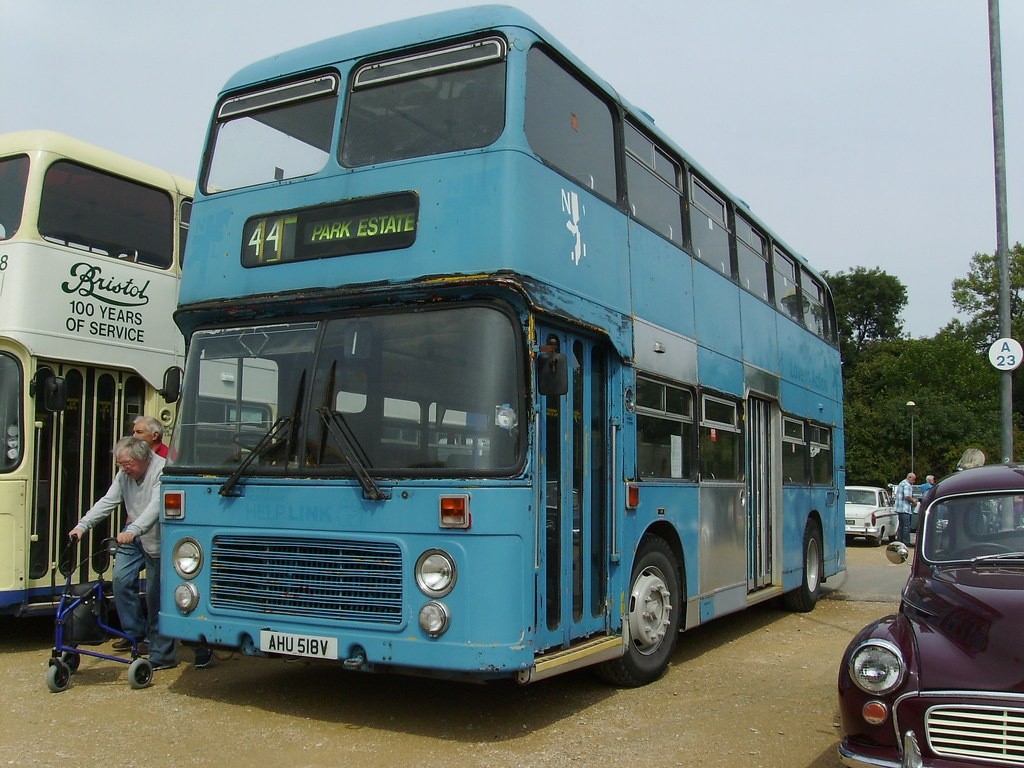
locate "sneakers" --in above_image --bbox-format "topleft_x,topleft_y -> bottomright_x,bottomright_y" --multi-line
136,643 -> 150,655
148,657 -> 181,671
193,645 -> 215,667
111,638 -> 134,651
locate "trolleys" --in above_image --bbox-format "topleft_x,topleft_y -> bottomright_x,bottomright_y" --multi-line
46,534 -> 153,691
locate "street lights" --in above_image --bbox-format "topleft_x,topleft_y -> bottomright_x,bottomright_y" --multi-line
905,399 -> 917,473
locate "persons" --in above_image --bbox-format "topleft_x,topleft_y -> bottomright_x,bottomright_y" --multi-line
894,473 -> 916,547
921,475 -> 934,494
948,448 -> 1024,551
68,416 -> 233,671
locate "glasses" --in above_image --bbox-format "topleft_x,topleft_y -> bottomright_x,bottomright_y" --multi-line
114,458 -> 135,469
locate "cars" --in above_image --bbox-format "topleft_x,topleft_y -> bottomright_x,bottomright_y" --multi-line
837,461 -> 1024,768
844,486 -> 900,547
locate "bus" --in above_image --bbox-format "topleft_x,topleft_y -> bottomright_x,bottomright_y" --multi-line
155,3 -> 849,689
0,130 -> 477,644
